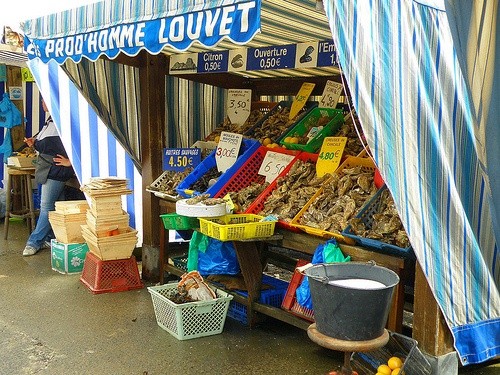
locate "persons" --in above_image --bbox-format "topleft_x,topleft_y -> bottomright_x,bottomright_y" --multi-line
22,98 -> 74,257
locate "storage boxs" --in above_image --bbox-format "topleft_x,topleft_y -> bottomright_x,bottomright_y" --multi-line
147,281 -> 234,341
347,328 -> 434,375
80,196 -> 144,294
48,201 -> 90,275
145,102 -> 415,326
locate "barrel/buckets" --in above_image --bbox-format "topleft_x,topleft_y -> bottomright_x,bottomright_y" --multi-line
295,260 -> 400,342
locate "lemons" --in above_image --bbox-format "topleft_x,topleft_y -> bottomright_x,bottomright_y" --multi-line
375,356 -> 405,375
262,137 -> 298,148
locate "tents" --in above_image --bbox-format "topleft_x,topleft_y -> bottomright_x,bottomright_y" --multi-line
0,0 -> 500,375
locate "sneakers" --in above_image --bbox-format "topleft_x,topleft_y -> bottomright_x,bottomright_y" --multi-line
41,240 -> 51,248
23,246 -> 39,255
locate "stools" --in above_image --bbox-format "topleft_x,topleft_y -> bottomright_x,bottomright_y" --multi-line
4,167 -> 34,239
307,322 -> 389,375
